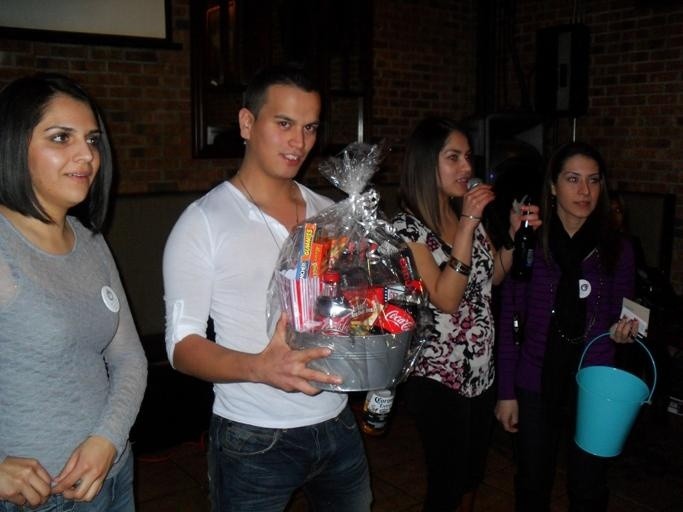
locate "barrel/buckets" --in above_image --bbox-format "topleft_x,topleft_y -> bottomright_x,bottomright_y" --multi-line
574,331 -> 658,458
291,330 -> 425,391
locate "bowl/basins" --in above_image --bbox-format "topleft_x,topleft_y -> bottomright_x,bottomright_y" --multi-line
283,319 -> 412,392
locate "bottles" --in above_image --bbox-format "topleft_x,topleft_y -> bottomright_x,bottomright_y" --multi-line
313,270 -> 351,335
368,279 -> 423,335
359,386 -> 395,436
510,198 -> 536,281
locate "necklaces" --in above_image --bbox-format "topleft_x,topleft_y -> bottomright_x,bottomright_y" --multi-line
234,168 -> 301,254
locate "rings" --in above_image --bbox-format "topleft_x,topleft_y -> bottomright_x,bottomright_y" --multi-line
629,333 -> 637,341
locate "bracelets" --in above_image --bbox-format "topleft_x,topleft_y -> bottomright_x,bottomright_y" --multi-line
446,254 -> 472,277
497,245 -> 510,278
459,213 -> 482,222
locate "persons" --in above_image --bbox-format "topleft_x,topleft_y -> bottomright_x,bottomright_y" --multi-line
381,112 -> 542,512
162,60 -> 376,511
0,71 -> 151,510
489,139 -> 641,512
